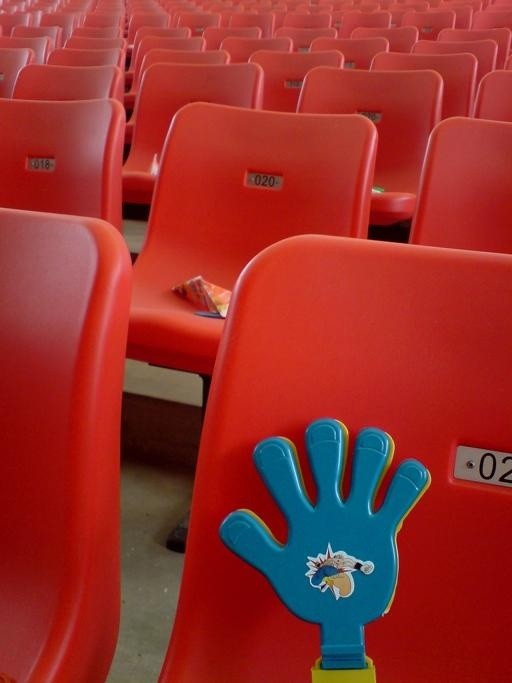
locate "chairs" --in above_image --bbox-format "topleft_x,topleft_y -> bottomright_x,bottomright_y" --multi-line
155,228 -> 512,682
409,115 -> 512,256
0,204 -> 140,682
127,99 -> 383,554
0,0 -> 512,240
